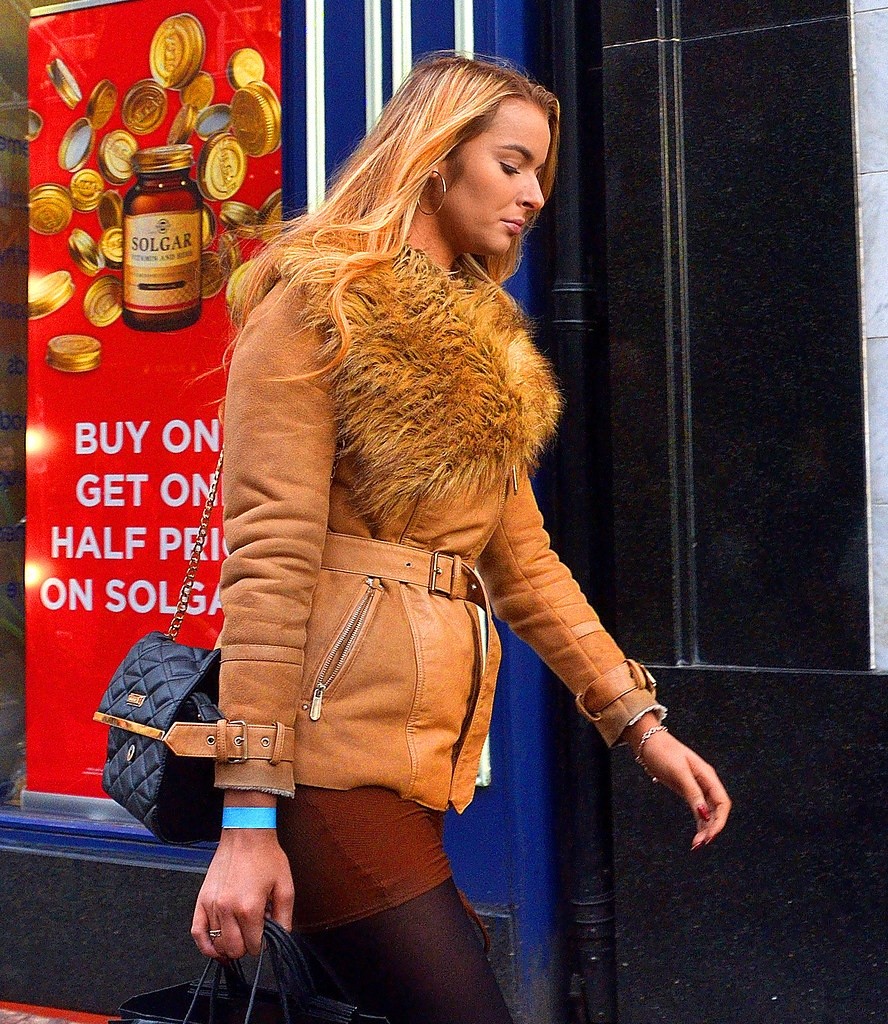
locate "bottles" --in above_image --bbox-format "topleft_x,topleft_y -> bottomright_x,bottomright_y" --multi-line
123,143 -> 205,330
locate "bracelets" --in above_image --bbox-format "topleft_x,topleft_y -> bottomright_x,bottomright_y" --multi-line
221,806 -> 277,829
635,725 -> 669,784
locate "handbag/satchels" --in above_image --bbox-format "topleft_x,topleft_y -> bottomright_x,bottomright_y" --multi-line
105,916 -> 389,1024
92,631 -> 222,843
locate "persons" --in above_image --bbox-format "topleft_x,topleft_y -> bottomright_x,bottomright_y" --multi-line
191,50 -> 733,1024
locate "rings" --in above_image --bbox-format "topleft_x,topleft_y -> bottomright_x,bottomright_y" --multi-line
210,929 -> 221,937
